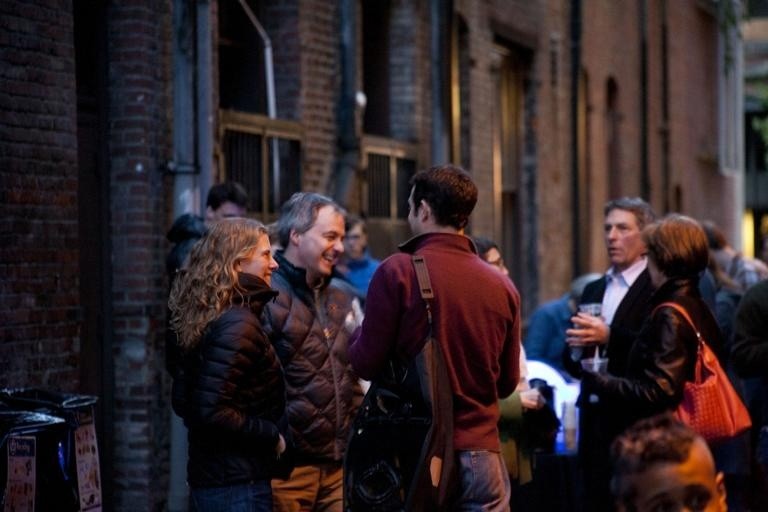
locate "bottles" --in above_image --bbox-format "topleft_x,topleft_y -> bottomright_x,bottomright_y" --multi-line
562,400 -> 577,450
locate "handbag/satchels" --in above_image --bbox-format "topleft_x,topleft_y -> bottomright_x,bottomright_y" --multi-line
671,344 -> 751,444
343,384 -> 447,511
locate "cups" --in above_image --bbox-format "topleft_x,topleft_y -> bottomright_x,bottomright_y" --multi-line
582,355 -> 609,403
577,303 -> 604,319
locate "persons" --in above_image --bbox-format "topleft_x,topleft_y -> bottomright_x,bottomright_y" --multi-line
730,277 -> 767,369
160,179 -> 254,425
692,215 -> 767,296
255,190 -> 364,512
564,194 -> 660,416
470,235 -> 546,512
607,414 -> 728,512
558,211 -> 752,512
166,216 -> 288,512
343,161 -> 521,512
331,208 -> 384,305
522,270 -> 604,383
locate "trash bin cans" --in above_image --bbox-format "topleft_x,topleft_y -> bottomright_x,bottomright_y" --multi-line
0,389 -> 103,512
